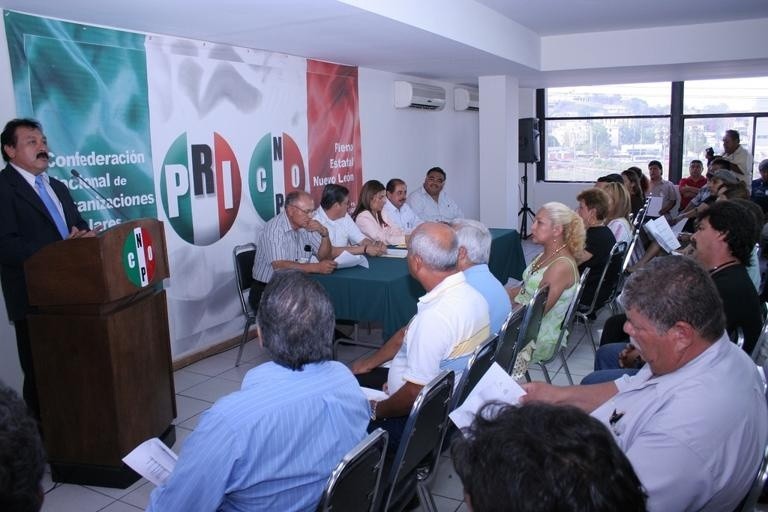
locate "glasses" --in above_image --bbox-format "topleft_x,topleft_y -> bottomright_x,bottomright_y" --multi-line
285,204 -> 317,216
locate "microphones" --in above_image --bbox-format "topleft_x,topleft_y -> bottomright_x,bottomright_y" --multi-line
70,169 -> 130,220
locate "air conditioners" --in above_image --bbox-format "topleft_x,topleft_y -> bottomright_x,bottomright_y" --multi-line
454,86 -> 478,111
394,81 -> 447,111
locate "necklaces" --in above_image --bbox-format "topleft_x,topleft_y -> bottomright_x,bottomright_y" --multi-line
532,244 -> 567,272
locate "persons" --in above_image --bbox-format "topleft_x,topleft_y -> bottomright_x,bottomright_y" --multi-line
366,222 -> 488,435
580,200 -> 764,386
599,128 -> 768,347
249,191 -> 337,316
352,219 -> 510,392
352,180 -> 407,245
505,202 -> 586,365
381,179 -> 423,235
1,117 -> 104,458
520,255 -> 768,512
576,160 -> 677,311
451,397 -> 649,512
0,378 -> 46,512
144,266 -> 371,512
407,166 -> 464,223
312,184 -> 387,257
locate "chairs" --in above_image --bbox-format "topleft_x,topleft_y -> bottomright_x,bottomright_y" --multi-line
497,194 -> 652,388
316,426 -> 390,512
751,303 -> 767,361
232,243 -> 256,366
740,454 -> 768,511
442,333 -> 501,458
378,366 -> 456,512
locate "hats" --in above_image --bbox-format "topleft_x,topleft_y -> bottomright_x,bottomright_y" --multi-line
706,158 -> 738,185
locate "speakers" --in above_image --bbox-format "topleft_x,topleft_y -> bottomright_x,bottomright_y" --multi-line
519,118 -> 540,162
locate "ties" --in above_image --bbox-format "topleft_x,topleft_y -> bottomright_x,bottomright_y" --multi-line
35,176 -> 70,241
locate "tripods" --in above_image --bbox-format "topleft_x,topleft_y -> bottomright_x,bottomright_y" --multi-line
518,162 -> 536,240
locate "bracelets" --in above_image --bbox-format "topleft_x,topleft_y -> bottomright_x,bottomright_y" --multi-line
364,245 -> 368,253
321,232 -> 329,237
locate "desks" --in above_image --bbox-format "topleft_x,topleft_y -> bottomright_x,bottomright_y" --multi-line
299,229 -> 527,365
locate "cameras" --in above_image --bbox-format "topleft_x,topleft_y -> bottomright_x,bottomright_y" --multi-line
705,147 -> 714,156
